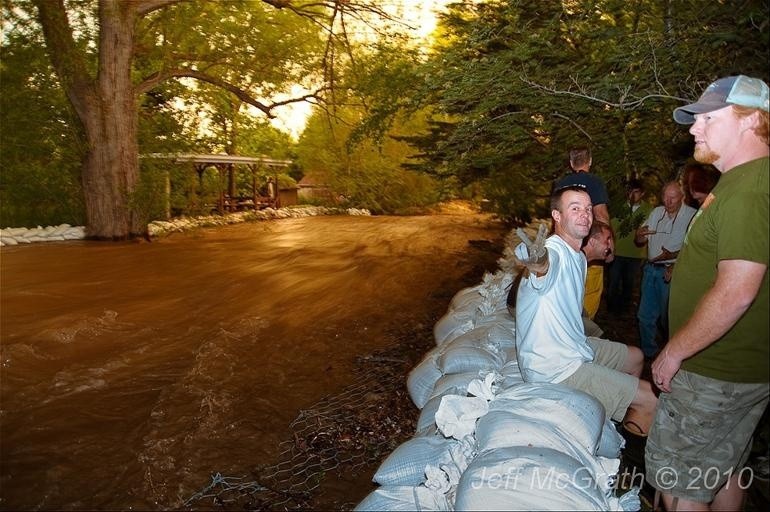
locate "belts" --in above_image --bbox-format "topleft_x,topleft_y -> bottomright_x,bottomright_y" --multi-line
648,261 -> 671,267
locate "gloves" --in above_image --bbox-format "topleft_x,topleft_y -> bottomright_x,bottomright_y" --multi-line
514,223 -> 549,266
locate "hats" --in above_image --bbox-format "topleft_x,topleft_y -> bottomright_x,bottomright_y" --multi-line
673,75 -> 770,125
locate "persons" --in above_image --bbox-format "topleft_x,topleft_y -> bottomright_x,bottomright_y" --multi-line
644,75 -> 770,511
508,144 -> 718,510
